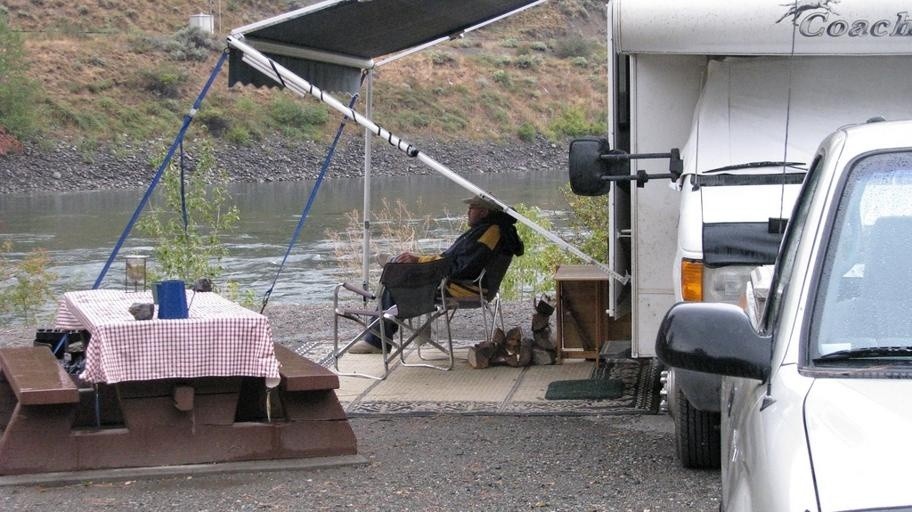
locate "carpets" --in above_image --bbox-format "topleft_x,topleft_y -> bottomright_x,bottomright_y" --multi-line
289,337 -> 661,418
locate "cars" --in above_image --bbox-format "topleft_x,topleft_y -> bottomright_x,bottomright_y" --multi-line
655,120 -> 912,511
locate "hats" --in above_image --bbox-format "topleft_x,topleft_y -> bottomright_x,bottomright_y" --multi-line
461,192 -> 497,211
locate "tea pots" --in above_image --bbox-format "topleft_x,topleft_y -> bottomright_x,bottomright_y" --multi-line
155,278 -> 195,320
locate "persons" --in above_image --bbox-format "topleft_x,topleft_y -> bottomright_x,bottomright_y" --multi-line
348,195 -> 524,354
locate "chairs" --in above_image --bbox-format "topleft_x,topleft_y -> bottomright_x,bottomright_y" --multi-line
334,236 -> 517,380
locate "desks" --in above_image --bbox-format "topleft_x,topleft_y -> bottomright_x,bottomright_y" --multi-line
59,286 -> 270,473
553,262 -> 613,368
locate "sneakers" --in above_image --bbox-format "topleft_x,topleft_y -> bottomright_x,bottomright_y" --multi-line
376,253 -> 396,269
347,340 -> 382,354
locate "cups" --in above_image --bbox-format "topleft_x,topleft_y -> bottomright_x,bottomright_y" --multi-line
152,281 -> 163,304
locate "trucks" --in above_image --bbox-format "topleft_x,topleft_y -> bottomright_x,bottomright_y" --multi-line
568,0 -> 912,469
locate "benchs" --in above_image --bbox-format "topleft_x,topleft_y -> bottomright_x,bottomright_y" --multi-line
1,345 -> 80,478
272,342 -> 356,458
